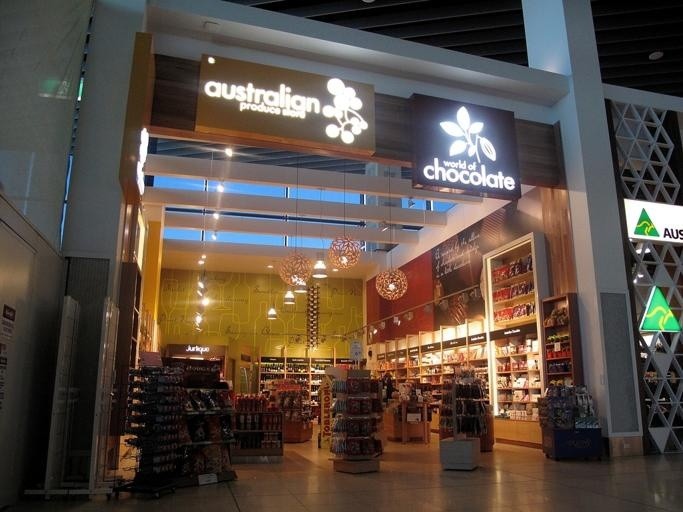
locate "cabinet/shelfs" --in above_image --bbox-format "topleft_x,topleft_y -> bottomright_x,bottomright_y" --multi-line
439,378 -> 494,451
255,348 -> 358,416
128,345 -> 237,487
331,379 -> 383,472
231,395 -> 284,463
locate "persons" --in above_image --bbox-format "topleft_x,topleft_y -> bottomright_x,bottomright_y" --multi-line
381,373 -> 398,401
